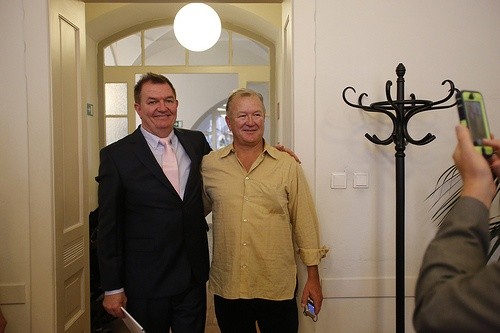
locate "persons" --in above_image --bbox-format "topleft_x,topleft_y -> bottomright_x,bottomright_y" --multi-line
98,72 -> 302,333
412,124 -> 500,333
200,88 -> 328,333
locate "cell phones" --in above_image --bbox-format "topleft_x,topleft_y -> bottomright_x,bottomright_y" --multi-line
456,90 -> 494,155
303,297 -> 318,323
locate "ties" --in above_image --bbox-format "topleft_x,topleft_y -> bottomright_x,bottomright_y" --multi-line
159,136 -> 180,197
